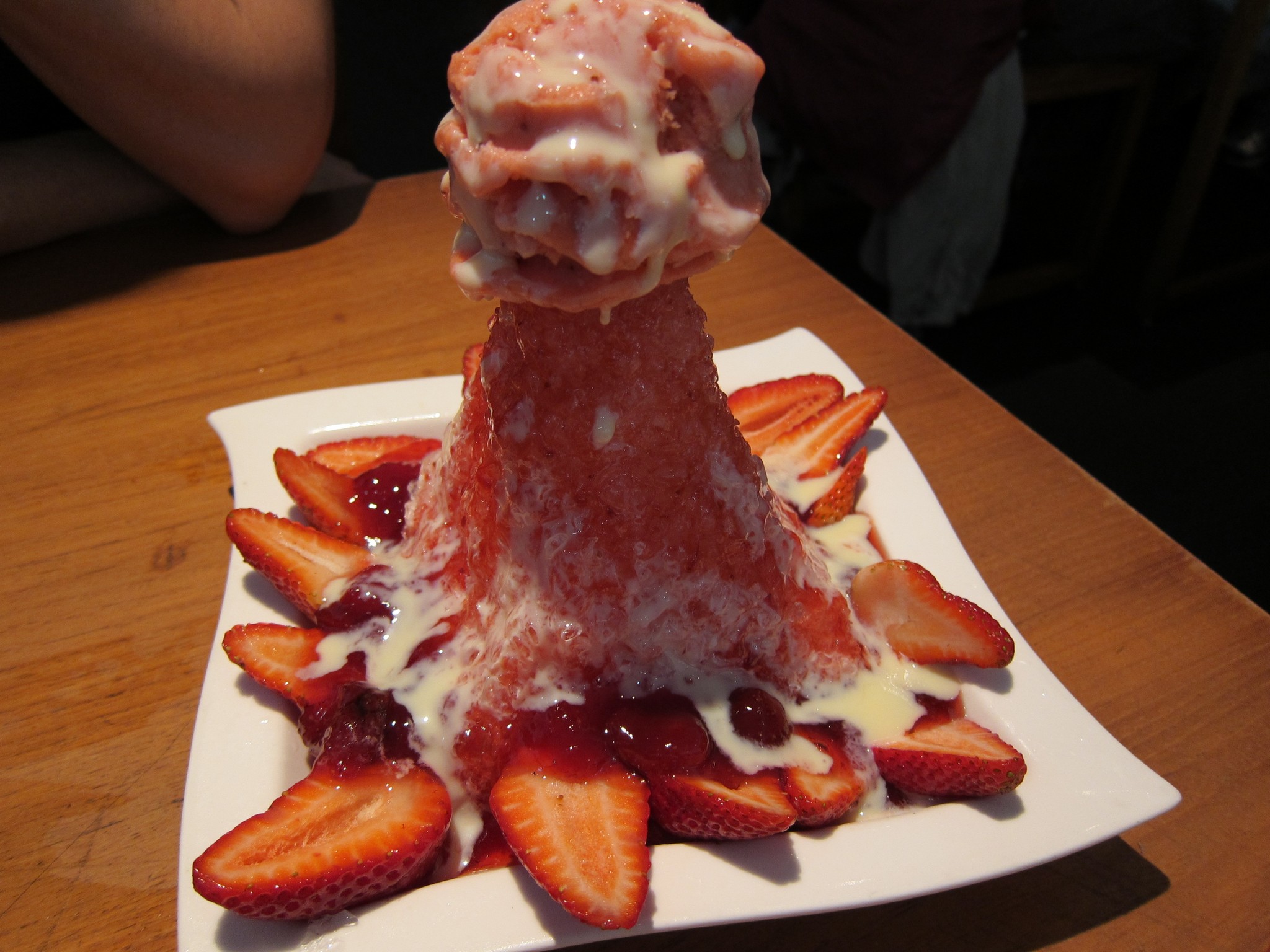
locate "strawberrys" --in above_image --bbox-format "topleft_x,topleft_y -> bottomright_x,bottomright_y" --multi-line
194,372 -> 1029,933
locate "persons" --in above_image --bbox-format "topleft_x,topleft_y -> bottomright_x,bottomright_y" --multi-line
2,2 -> 342,249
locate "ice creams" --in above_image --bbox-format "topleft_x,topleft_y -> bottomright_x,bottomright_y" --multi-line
299,0 -> 951,869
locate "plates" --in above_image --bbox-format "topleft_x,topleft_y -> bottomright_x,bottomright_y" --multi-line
171,317 -> 1182,952
